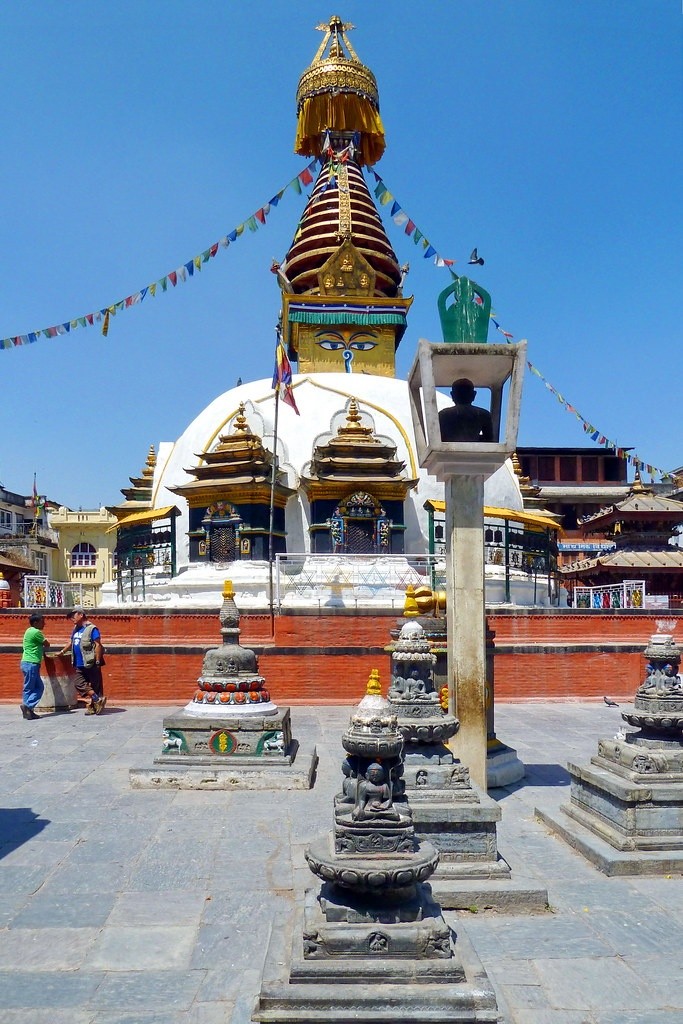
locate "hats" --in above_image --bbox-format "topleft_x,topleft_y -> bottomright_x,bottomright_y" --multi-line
67,605 -> 85,618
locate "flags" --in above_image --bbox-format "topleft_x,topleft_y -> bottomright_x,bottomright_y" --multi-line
31,479 -> 44,522
271,333 -> 300,415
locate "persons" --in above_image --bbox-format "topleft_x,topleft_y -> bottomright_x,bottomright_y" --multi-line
439,379 -> 494,443
55,608 -> 107,715
20,614 -> 50,720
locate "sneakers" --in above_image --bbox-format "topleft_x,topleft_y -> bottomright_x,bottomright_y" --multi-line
85,705 -> 96,715
93,697 -> 107,714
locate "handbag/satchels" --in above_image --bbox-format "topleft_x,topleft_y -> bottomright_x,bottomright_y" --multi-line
85,651 -> 105,668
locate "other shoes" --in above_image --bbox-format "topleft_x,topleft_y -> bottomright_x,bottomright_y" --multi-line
20,704 -> 32,720
23,710 -> 40,719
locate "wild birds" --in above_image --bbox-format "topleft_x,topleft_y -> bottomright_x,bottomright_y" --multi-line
467,247 -> 484,266
236,377 -> 242,386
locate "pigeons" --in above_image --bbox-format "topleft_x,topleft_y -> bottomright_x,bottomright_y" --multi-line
603,696 -> 619,707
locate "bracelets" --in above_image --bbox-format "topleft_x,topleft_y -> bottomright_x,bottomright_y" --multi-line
61,650 -> 65,654
